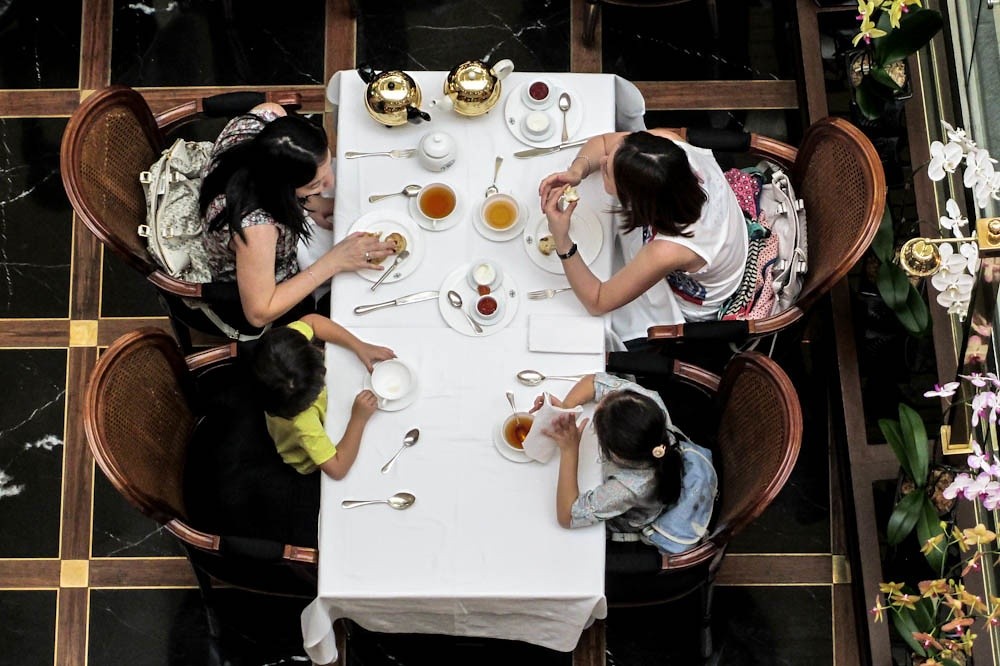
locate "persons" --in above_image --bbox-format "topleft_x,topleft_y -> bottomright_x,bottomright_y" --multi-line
538,128 -> 749,322
244,314 -> 398,480
198,102 -> 395,328
530,371 -> 685,554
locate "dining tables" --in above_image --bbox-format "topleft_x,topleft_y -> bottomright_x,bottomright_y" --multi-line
301,71 -> 647,666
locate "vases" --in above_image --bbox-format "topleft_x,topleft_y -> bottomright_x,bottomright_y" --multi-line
890,461 -> 958,565
855,258 -> 926,334
841,50 -> 912,119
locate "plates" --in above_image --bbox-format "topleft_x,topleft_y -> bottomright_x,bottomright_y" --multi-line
364,359 -> 421,412
438,263 -> 519,337
348,210 -> 424,284
494,408 -> 539,463
504,77 -> 584,149
521,79 -> 556,111
520,113 -> 556,142
523,203 -> 604,275
471,191 -> 529,242
408,193 -> 466,232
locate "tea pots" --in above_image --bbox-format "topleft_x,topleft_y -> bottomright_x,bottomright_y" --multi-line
357,61 -> 432,129
430,59 -> 515,116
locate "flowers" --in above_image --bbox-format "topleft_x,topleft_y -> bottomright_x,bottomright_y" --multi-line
843,1 -> 999,666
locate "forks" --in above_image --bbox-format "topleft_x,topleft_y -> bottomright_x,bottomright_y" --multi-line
345,149 -> 417,160
526,287 -> 572,299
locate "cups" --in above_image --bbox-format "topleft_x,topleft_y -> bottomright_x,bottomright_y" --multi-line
527,80 -> 550,104
370,360 -> 412,408
526,110 -> 551,134
417,182 -> 458,228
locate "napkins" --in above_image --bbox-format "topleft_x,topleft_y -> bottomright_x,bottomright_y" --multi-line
297,157 -> 336,309
609,221 -> 686,353
522,393 -> 583,463
528,313 -> 603,355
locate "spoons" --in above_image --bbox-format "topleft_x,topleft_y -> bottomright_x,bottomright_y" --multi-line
507,392 -> 528,441
516,370 -> 584,386
485,156 -> 503,198
448,291 -> 483,335
368,184 -> 422,204
559,92 -> 571,142
342,492 -> 416,511
381,428 -> 419,475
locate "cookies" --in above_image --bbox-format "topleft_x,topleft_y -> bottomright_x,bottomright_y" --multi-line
360,233 -> 387,265
538,236 -> 557,256
385,232 -> 406,255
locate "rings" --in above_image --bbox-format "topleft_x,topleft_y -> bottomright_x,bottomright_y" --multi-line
366,252 -> 368,257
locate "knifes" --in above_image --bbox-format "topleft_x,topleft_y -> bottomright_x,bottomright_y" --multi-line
354,290 -> 440,316
370,250 -> 409,291
513,137 -> 588,159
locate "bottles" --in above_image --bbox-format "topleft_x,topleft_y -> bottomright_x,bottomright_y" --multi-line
417,130 -> 459,172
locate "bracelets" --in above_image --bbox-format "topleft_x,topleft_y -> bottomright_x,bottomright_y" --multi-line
572,155 -> 592,179
556,244 -> 577,259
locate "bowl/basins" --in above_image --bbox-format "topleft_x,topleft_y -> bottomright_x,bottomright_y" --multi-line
501,411 -> 535,452
479,193 -> 519,232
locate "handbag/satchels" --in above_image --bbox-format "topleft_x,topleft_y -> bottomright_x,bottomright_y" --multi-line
756,171 -> 807,313
136,137 -> 218,279
642,439 -> 718,555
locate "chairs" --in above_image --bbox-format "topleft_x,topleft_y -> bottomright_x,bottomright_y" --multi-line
82,325 -> 322,662
605,351 -> 804,657
645,115 -> 885,342
59,85 -> 301,353
